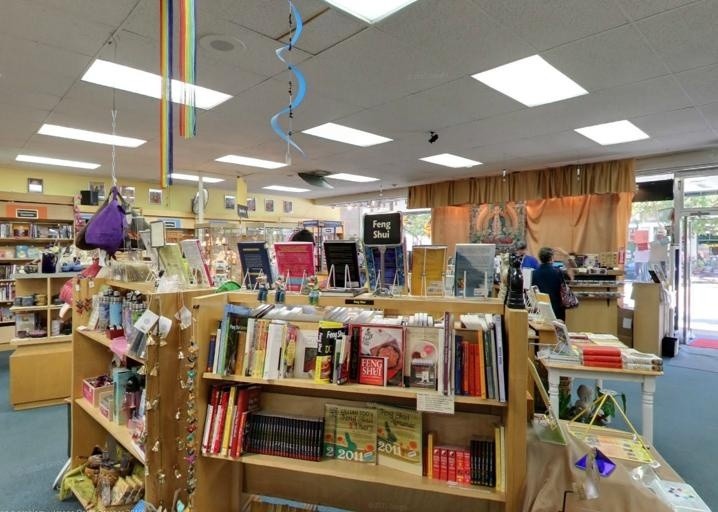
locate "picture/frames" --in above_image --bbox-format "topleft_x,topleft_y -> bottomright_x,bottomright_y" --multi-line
26,176 -> 293,213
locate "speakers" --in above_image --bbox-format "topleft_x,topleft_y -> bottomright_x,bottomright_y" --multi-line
80,189 -> 98,205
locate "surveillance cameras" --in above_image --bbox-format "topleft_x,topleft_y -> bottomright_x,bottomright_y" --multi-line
428,132 -> 438,143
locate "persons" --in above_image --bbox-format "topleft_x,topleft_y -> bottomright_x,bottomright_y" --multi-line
510,239 -> 537,267
483,202 -> 514,245
532,246 -> 570,325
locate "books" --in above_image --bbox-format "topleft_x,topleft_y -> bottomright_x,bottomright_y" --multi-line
181,236 -> 216,287
322,238 -> 363,289
200,385 -> 506,493
578,342 -> 664,373
363,236 -> 409,295
273,240 -> 316,292
201,303 -> 507,403
236,240 -> 275,292
156,241 -> 187,285
451,242 -> 496,299
410,243 -> 448,294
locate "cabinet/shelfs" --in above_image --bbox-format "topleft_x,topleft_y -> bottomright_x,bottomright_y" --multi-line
0,193 -> 713,512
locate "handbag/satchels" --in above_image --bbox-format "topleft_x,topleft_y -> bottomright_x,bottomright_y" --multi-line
560,279 -> 579,308
75,199 -> 127,255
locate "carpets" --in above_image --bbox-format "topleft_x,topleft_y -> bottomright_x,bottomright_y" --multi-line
688,338 -> 718,350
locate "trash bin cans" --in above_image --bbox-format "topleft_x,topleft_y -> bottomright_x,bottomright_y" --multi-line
662,337 -> 679,358
